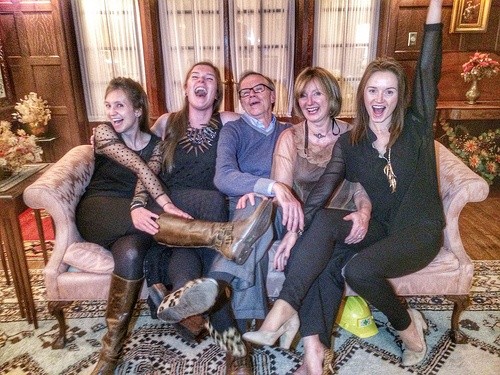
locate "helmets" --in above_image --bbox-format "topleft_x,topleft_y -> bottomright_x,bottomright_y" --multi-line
335,295 -> 379,338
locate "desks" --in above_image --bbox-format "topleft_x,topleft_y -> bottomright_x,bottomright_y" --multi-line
37,135 -> 61,164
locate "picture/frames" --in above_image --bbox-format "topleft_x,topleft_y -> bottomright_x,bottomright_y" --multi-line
449,0 -> 492,33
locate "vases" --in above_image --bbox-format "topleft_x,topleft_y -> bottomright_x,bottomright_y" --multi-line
30,121 -> 47,136
465,77 -> 481,105
0,164 -> 13,180
492,175 -> 500,189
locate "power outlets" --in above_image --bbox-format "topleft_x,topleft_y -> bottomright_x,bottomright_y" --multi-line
407,32 -> 418,46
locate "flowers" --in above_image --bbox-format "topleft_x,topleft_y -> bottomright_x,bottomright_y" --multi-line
460,51 -> 500,82
0,121 -> 44,171
12,93 -> 53,126
440,113 -> 499,185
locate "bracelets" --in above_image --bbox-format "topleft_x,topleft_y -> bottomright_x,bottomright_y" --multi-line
296,229 -> 304,237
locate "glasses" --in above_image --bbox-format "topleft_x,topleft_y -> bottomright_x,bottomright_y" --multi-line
238,83 -> 273,99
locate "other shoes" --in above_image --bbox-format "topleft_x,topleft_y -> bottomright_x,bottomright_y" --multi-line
147,283 -> 206,342
204,321 -> 247,357
156,277 -> 219,324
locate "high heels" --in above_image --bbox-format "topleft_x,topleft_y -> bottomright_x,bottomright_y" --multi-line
320,343 -> 335,375
242,311 -> 301,350
402,308 -> 428,367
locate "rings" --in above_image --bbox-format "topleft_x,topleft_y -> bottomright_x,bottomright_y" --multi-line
358,235 -> 362,239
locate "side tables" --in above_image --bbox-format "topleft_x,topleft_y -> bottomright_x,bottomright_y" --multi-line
0,162 -> 55,329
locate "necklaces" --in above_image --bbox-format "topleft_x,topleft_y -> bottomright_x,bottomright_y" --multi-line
309,125 -> 331,139
372,142 -> 397,193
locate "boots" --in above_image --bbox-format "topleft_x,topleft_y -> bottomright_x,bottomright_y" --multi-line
89,271 -> 145,375
154,198 -> 273,266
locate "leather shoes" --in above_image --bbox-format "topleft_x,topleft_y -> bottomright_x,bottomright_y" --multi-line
225,340 -> 254,375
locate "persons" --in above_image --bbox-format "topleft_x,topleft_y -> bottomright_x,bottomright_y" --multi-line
242,0 -> 455,366
76,62 -> 372,375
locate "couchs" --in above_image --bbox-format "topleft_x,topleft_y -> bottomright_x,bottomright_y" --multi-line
24,135 -> 489,343
